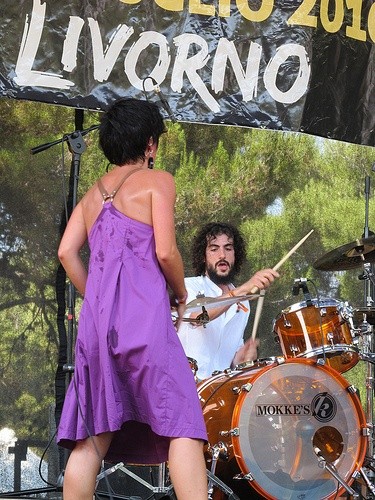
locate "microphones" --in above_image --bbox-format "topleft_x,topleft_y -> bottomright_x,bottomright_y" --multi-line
292,279 -> 300,295
152,79 -> 177,124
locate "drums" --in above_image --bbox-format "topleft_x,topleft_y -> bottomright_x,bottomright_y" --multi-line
196,357 -> 366,500
273,297 -> 360,373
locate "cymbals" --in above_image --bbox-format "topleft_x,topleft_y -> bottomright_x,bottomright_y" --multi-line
186,295 -> 260,311
315,235 -> 375,271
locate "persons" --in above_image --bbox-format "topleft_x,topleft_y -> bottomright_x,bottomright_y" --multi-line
168,222 -> 282,372
50,97 -> 214,500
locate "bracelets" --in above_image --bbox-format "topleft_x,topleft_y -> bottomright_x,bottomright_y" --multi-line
227,289 -> 249,312
174,291 -> 188,304
